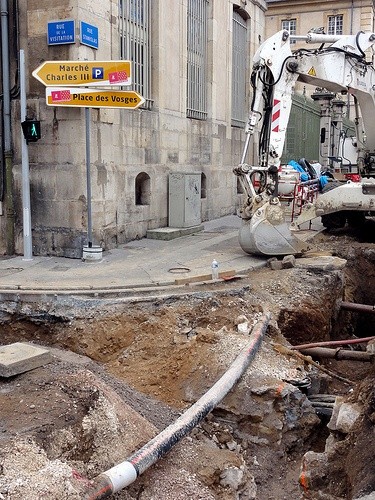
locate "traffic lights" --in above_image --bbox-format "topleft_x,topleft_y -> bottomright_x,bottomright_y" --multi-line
20,120 -> 42,142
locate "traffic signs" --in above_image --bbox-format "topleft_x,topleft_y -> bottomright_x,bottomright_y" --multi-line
46,86 -> 147,109
31,60 -> 133,87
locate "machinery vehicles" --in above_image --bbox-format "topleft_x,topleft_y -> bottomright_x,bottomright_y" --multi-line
232,28 -> 375,255
